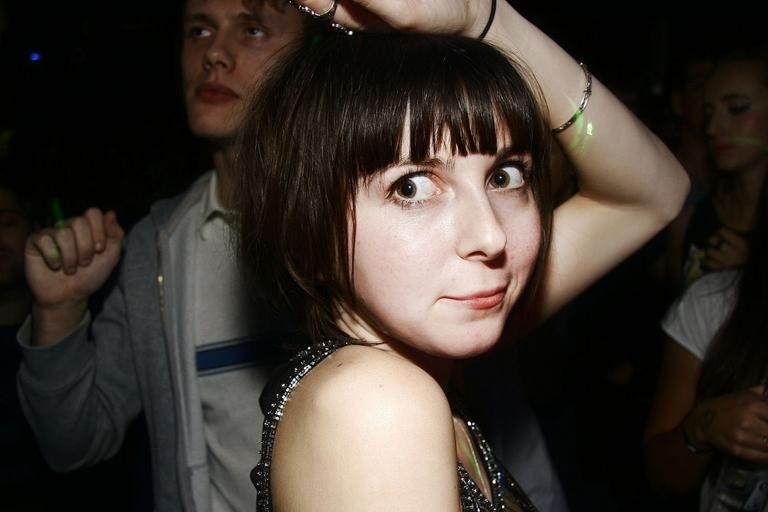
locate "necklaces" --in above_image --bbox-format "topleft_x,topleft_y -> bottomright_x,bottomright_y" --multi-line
453,418 -> 492,495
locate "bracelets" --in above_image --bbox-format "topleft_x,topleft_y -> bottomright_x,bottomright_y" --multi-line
479,0 -> 497,42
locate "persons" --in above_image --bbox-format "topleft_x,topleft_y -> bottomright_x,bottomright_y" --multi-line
16,2 -> 327,512
529,48 -> 768,511
232,0 -> 691,511
0,158 -> 144,511
